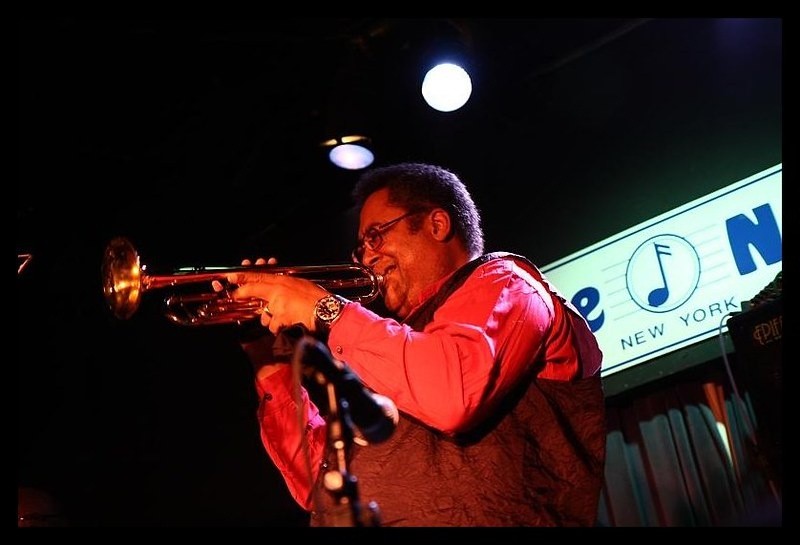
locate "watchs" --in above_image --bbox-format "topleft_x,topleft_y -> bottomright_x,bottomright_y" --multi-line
313,294 -> 352,346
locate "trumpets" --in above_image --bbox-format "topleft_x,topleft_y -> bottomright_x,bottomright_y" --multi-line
101,236 -> 390,329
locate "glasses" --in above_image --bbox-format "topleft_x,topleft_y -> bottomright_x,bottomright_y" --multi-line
352,209 -> 430,263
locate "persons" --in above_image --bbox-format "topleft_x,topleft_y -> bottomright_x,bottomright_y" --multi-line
211,161 -> 607,528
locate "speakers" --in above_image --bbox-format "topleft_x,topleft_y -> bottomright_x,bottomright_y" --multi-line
725,297 -> 785,496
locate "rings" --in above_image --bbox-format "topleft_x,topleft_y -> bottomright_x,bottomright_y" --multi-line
264,304 -> 273,317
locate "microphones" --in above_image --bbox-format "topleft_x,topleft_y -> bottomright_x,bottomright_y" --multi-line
300,339 -> 400,444
